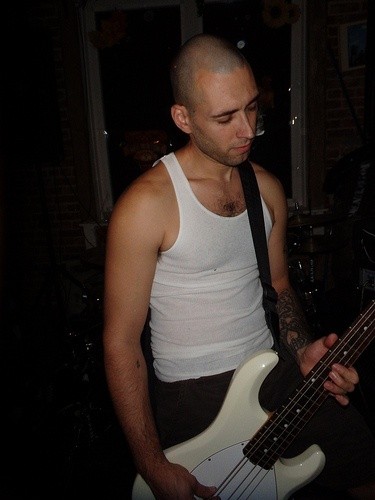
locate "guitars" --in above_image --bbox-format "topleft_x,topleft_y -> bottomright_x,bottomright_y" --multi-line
131,293 -> 375,500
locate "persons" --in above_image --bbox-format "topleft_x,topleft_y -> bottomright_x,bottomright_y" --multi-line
102,33 -> 359,500
